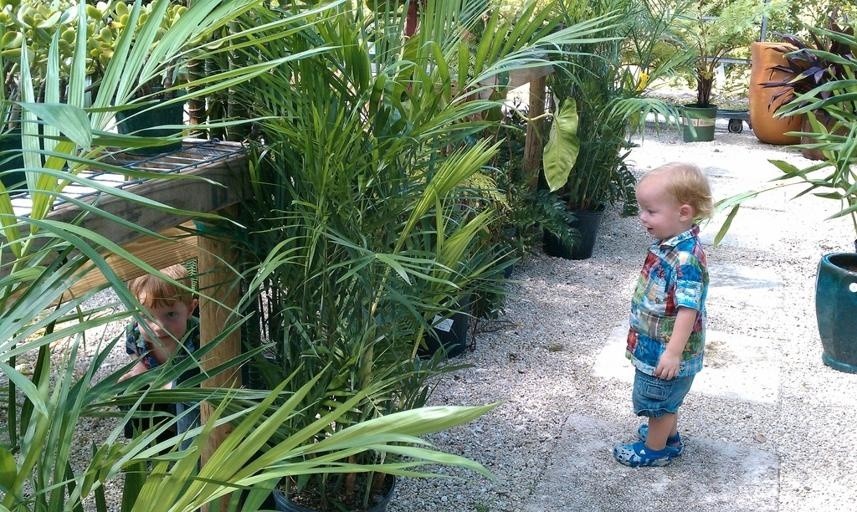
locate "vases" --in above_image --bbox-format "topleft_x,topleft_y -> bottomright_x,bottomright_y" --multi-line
748,39 -> 801,146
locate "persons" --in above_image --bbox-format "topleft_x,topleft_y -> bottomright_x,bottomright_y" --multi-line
611,161 -> 715,468
111,262 -> 200,453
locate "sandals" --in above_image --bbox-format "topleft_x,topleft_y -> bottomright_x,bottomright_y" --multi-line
635,421 -> 684,457
611,437 -> 673,469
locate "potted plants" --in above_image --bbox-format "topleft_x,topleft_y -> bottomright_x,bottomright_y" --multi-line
1,0 -> 753,512
747,4 -> 854,372
754,12 -> 855,162
